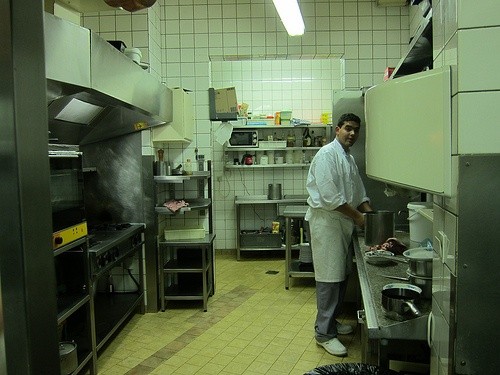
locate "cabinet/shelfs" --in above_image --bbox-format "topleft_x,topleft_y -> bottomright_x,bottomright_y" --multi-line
233,195 -> 309,262
154,158 -> 217,312
54,221 -> 149,375
283,205 -> 315,290
222,122 -> 332,169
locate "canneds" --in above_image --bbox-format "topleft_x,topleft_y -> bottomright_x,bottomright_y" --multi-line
246,155 -> 252,165
314,136 -> 326,147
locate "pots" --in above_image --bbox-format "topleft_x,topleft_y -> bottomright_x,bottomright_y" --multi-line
381,288 -> 422,320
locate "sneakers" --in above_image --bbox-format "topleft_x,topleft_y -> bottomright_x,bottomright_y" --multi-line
335,323 -> 353,334
315,336 -> 347,355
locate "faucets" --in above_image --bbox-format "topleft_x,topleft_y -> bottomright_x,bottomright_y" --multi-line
398,208 -> 408,216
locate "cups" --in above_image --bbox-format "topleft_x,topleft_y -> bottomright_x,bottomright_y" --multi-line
156,161 -> 173,176
275,157 -> 283,164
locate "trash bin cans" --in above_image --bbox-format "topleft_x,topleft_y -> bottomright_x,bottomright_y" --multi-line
304,363 -> 403,375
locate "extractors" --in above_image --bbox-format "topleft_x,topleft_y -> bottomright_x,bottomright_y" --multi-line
43,10 -> 173,146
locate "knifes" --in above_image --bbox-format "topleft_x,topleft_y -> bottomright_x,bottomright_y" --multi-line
376,274 -> 410,280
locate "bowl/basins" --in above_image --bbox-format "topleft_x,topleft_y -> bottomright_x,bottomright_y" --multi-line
364,251 -> 394,265
405,270 -> 432,292
403,247 -> 433,277
172,170 -> 186,175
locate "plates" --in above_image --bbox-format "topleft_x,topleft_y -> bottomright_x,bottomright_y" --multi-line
383,283 -> 422,293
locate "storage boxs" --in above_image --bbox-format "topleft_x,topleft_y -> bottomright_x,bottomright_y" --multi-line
240,230 -> 282,248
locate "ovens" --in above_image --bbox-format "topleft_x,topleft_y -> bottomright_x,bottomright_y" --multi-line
48,148 -> 88,234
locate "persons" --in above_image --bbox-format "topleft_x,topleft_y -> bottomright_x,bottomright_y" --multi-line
305,113 -> 373,357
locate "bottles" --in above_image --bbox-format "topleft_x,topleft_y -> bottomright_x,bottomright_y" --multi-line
246,156 -> 252,165
311,131 -> 326,147
305,128 -> 311,147
185,159 -> 193,175
275,112 -> 280,125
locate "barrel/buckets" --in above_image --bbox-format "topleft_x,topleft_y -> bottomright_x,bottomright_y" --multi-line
59,341 -> 79,375
268,184 -> 281,200
298,243 -> 312,263
407,202 -> 433,242
57,324 -> 63,341
363,209 -> 401,247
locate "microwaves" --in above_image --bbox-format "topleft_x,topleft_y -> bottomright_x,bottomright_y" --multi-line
226,130 -> 258,148
